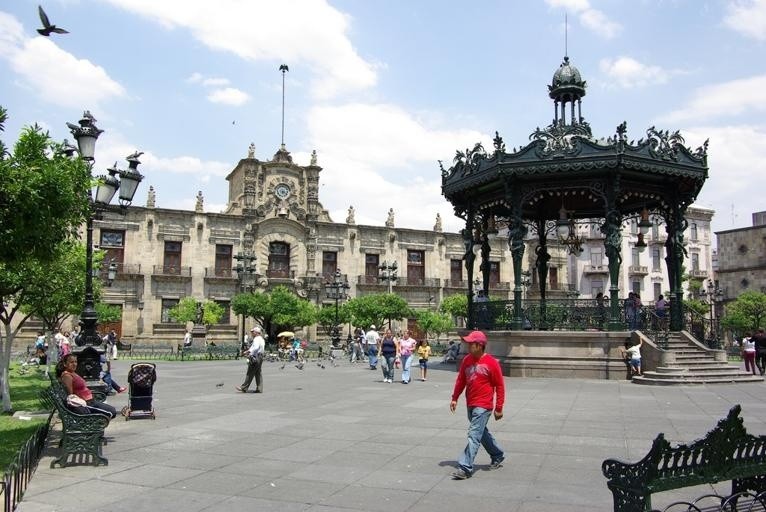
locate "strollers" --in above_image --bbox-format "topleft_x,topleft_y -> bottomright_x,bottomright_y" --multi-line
116,361 -> 157,422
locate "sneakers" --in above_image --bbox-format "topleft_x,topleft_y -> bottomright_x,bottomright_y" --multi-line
117,387 -> 126,393
452,470 -> 470,479
632,369 -> 642,375
236,386 -> 246,392
760,369 -> 764,375
488,455 -> 505,469
404,380 -> 408,384
384,378 -> 392,383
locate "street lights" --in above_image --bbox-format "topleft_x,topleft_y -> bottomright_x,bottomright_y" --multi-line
473,275 -> 482,295
427,294 -> 434,314
58,101 -> 148,402
519,268 -> 533,305
323,265 -> 351,360
228,250 -> 255,358
697,277 -> 724,350
377,258 -> 399,333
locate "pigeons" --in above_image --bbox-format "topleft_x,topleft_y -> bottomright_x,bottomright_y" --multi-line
264,355 -> 338,371
37,6 -> 71,36
215,382 -> 224,387
8,409 -> 14,416
8,363 -> 29,375
65,122 -> 79,132
85,110 -> 97,123
210,340 -> 216,345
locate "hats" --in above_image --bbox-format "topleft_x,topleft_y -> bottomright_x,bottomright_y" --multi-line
251,327 -> 261,334
370,325 -> 375,328
463,331 -> 487,345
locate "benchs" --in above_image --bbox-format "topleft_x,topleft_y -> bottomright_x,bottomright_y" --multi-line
602,405 -> 766,512
104,339 -> 131,356
48,371 -> 109,469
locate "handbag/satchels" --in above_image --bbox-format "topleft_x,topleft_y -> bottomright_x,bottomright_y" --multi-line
249,355 -> 256,365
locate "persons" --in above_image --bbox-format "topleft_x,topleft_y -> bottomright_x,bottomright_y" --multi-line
347,324 -> 457,383
734,327 -> 766,375
450,331 -> 504,478
184,331 -> 192,347
243,332 -> 307,362
622,336 -> 644,375
33,325 -> 127,420
597,292 -> 668,331
476,290 -> 490,311
236,327 -> 265,393
